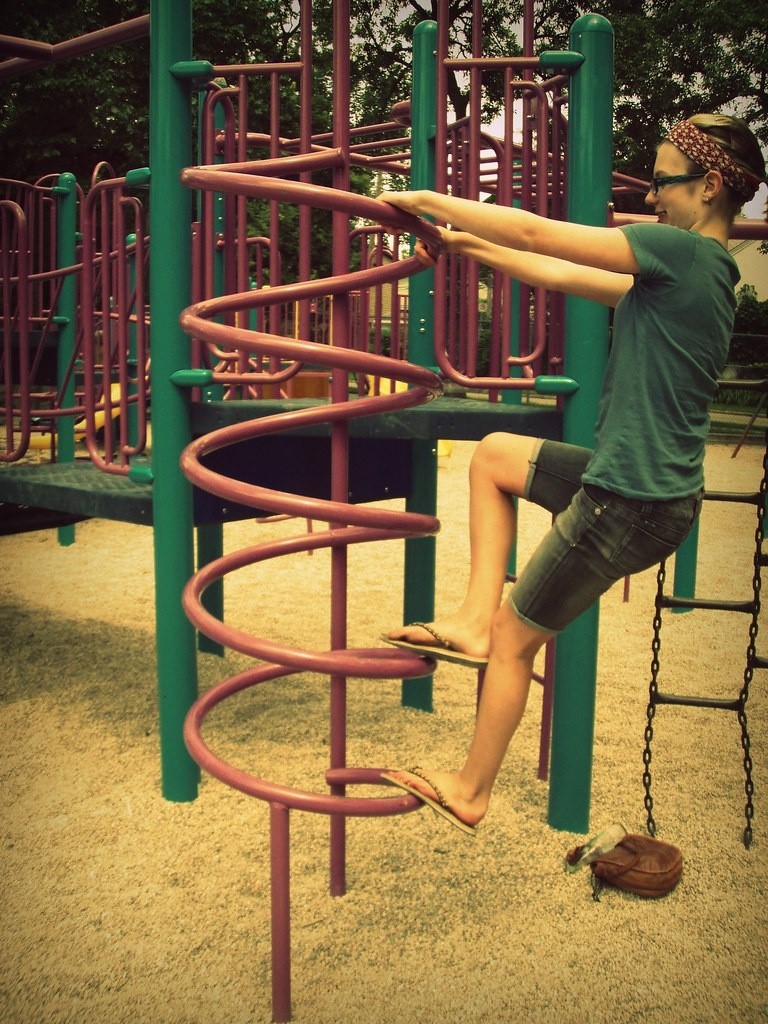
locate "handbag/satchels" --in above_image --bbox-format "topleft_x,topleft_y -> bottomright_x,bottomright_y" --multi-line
563,822 -> 683,897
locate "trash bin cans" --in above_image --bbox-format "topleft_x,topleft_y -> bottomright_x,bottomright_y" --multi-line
0,328 -> 60,421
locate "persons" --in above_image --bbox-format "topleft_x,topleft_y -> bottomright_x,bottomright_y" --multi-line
371,114 -> 766,837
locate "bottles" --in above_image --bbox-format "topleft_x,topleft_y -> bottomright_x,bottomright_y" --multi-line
566,823 -> 628,872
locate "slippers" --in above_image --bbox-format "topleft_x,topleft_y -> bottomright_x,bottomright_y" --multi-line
367,759 -> 477,836
379,622 -> 489,669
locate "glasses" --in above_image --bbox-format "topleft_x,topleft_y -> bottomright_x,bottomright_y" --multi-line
649,172 -> 717,195
